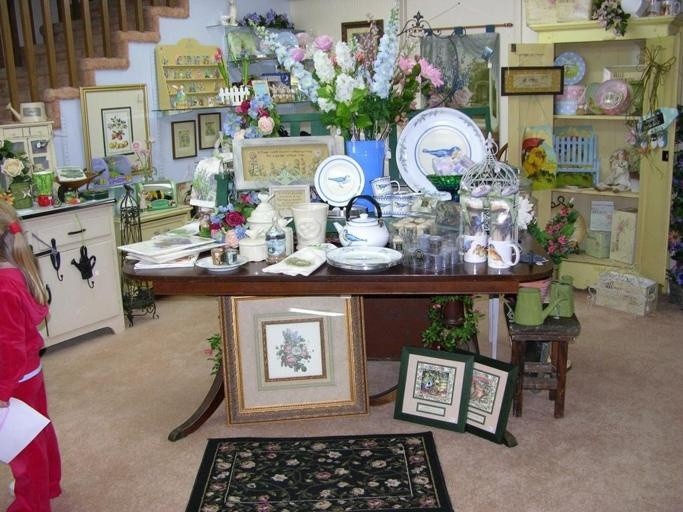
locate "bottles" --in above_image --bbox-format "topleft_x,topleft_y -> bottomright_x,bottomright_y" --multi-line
266,218 -> 293,264
210,248 -> 238,265
199,214 -> 210,239
177,84 -> 188,108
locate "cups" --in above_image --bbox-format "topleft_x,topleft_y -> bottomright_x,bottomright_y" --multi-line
291,202 -> 329,250
10,171 -> 54,209
369,175 -> 423,217
455,235 -> 520,269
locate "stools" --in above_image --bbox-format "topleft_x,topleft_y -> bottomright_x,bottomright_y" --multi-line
505,301 -> 581,418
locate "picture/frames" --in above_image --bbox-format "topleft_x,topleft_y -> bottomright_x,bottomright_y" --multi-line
217,290 -> 370,427
457,348 -> 519,444
500,66 -> 564,96
393,345 -> 474,432
232,135 -> 334,190
199,113 -> 222,149
79,82 -> 154,176
342,19 -> 385,43
171,120 -> 197,159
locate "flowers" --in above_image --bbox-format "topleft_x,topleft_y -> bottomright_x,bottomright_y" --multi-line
215,95 -> 275,162
530,199 -> 580,264
252,9 -> 446,140
0,139 -> 30,183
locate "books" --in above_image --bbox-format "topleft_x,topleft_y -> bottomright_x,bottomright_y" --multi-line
117,230 -> 226,269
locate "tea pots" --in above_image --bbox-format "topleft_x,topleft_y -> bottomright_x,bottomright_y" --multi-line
334,194 -> 389,247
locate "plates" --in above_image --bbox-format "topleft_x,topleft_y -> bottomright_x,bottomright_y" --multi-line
596,79 -> 628,110
116,232 -> 226,263
314,154 -> 365,208
396,106 -> 487,201
326,246 -> 402,273
197,256 -> 247,272
554,52 -> 586,85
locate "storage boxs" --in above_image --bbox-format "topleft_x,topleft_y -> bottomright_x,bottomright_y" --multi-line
610,208 -> 638,265
595,273 -> 659,317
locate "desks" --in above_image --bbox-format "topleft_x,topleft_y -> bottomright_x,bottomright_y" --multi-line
122,229 -> 553,447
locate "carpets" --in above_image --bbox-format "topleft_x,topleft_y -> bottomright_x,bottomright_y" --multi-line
184,431 -> 455,512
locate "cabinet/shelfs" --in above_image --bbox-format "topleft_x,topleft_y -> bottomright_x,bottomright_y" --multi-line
10,195 -> 125,348
155,37 -> 229,109
502,15 -> 682,295
114,204 -> 194,296
0,120 -> 58,179
206,23 -> 313,108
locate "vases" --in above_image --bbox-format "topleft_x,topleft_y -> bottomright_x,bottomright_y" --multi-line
346,141 -> 384,212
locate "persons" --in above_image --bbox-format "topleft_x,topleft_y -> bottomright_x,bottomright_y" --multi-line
0,198 -> 62,512
